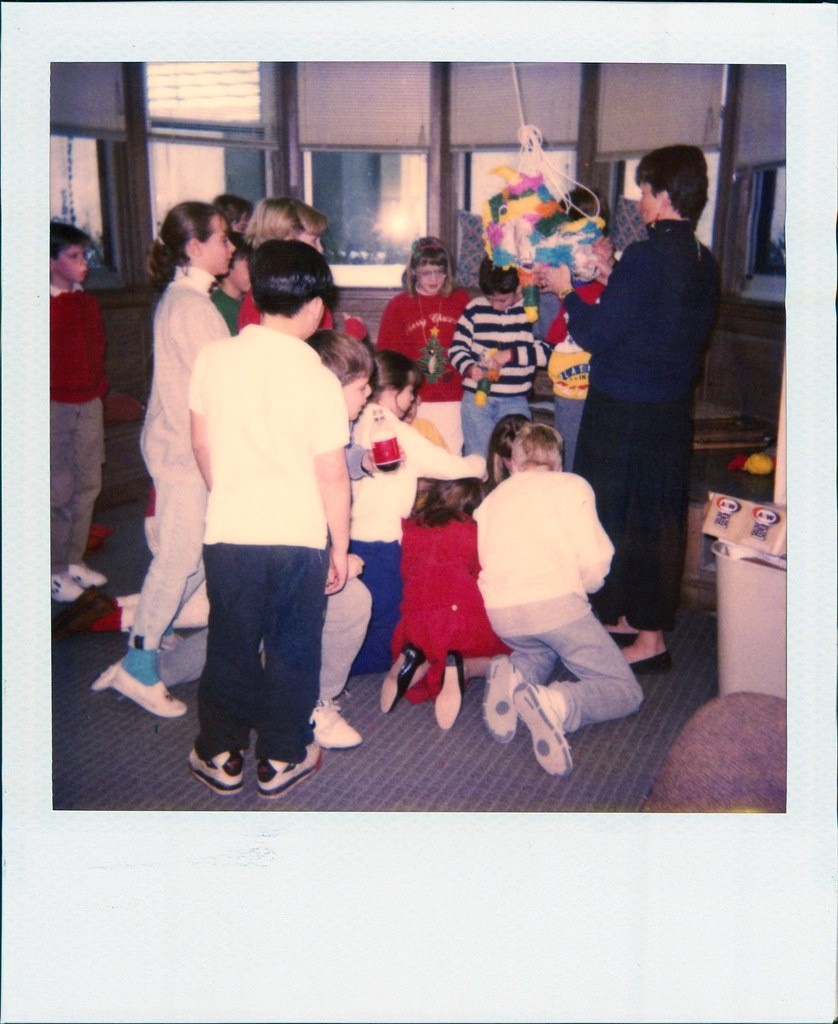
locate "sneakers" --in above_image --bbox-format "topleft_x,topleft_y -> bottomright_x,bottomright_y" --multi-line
308,697 -> 364,752
186,746 -> 246,795
255,740 -> 324,800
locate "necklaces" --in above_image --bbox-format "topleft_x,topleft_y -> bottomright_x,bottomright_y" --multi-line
418,291 -> 441,344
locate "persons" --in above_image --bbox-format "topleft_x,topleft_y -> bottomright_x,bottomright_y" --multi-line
535,142 -> 724,678
545,188 -> 624,473
470,420 -> 645,776
447,252 -> 551,463
348,351 -> 490,681
379,475 -> 514,729
184,239 -> 353,800
486,410 -> 524,478
377,236 -> 471,457
51,222 -> 113,602
95,191 -> 371,749
402,394 -> 445,512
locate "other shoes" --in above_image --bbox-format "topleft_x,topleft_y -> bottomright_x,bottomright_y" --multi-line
52,575 -> 84,603
112,667 -> 188,718
434,650 -> 465,730
91,659 -> 127,691
52,584 -> 119,639
381,644 -> 426,714
481,653 -> 525,744
619,642 -> 673,674
599,623 -> 638,649
513,683 -> 574,780
66,564 -> 108,587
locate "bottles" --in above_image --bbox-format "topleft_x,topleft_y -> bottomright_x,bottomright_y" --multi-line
369,408 -> 401,472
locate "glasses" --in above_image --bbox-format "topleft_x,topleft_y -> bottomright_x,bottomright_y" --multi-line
408,266 -> 447,279
